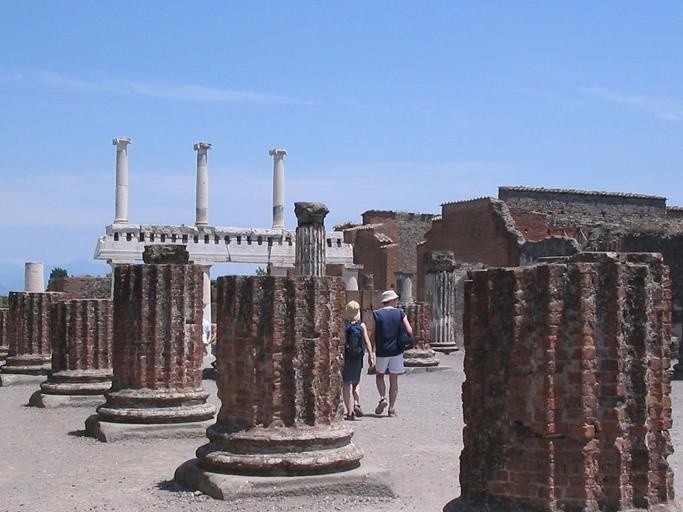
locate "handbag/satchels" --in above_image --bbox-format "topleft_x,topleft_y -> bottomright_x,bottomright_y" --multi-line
398,321 -> 414,352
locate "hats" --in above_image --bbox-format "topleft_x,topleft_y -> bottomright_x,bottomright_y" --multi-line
345,301 -> 360,321
380,290 -> 399,303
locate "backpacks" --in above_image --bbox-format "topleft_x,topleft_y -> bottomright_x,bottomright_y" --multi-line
344,321 -> 364,361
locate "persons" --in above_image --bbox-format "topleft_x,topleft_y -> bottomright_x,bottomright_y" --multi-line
340,299 -> 374,420
369,289 -> 413,417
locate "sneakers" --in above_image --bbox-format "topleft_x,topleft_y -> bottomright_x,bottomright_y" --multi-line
347,404 -> 362,420
388,409 -> 397,417
375,399 -> 388,414
